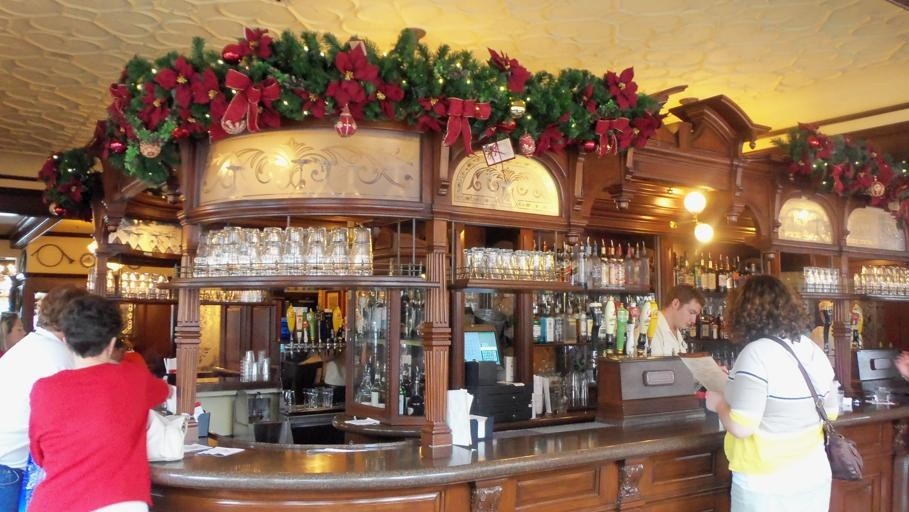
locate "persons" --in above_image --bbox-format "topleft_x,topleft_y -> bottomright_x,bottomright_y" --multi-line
122,337 -> 148,370
0,311 -> 28,358
894,350 -> 909,382
644,284 -> 706,356
704,274 -> 840,512
0,284 -> 96,512
111,342 -> 128,362
28,291 -> 170,512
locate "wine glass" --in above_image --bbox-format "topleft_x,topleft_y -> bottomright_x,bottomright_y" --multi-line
198,289 -> 264,303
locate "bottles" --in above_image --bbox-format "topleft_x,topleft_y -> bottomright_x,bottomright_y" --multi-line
280,303 -> 345,343
532,292 -> 657,353
353,258 -> 428,418
673,249 -> 761,363
534,235 -> 653,289
874,385 -> 891,404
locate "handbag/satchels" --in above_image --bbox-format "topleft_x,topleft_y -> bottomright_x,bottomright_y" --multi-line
144,406 -> 192,461
818,418 -> 868,483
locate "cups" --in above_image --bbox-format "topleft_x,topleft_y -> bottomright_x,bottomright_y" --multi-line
464,247 -> 554,280
107,271 -> 172,297
854,264 -> 908,295
194,225 -> 376,276
800,266 -> 841,291
563,371 -> 594,406
239,376 -> 272,384
240,350 -> 271,376
303,386 -> 336,409
282,389 -> 297,407
